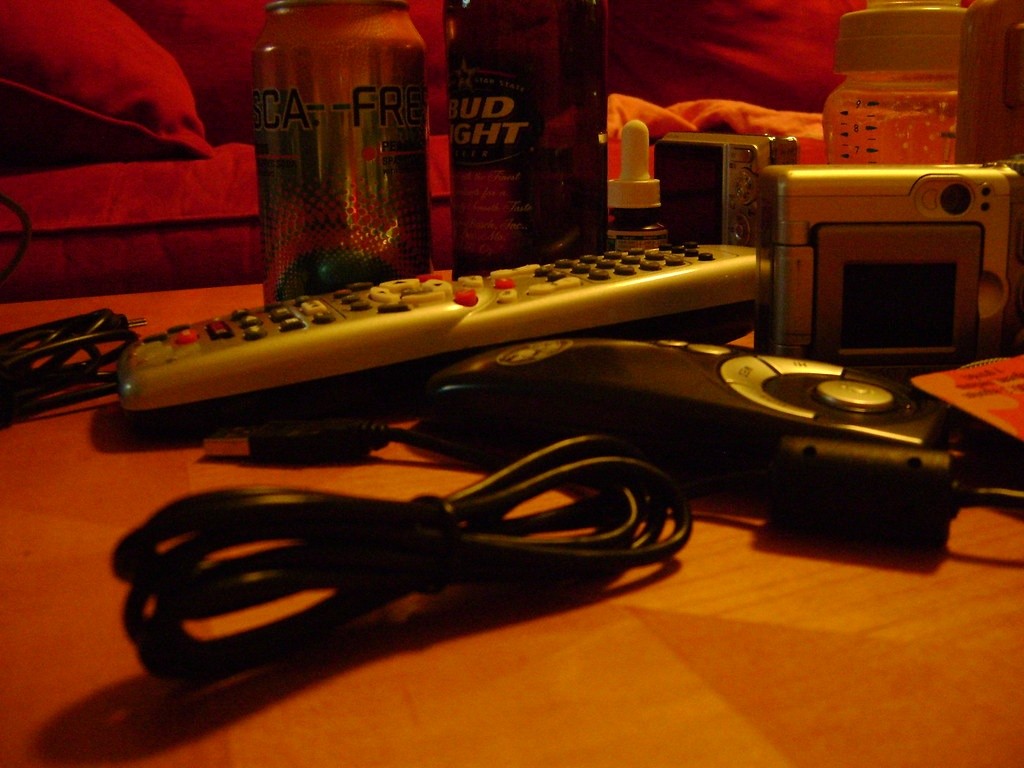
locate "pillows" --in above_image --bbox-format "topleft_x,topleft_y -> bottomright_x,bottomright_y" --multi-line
0,0 -> 213,159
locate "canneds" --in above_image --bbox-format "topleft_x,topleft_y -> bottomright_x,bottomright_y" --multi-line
443,0 -> 608,279
250,0 -> 430,305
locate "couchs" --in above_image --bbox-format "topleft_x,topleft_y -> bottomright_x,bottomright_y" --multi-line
0,0 -> 452,322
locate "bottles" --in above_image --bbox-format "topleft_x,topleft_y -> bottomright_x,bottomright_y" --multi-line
444,0 -> 608,282
822,0 -> 969,165
606,119 -> 668,251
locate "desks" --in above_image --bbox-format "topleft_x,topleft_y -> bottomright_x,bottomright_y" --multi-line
0,282 -> 1024,768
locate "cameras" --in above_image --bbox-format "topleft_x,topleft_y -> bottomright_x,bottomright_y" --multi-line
763,162 -> 1024,382
654,132 -> 797,249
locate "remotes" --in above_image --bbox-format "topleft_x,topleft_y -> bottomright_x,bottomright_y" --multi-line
433,335 -> 964,470
119,240 -> 772,448
